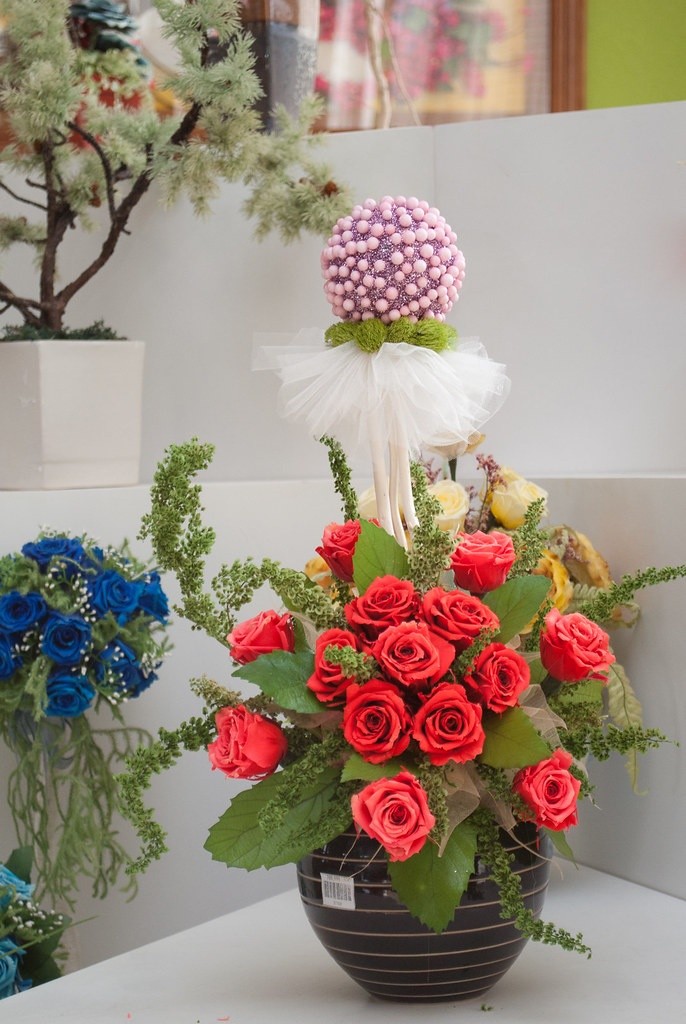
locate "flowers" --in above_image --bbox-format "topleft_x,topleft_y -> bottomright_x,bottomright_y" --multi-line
206,430 -> 686,955
0,844 -> 101,1001
0,526 -> 176,900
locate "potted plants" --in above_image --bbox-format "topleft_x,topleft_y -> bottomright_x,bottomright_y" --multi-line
0,0 -> 353,491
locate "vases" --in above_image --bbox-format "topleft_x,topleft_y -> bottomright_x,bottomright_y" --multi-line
298,809 -> 554,1004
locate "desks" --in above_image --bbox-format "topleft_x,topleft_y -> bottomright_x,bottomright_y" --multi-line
0,850 -> 686,1024
